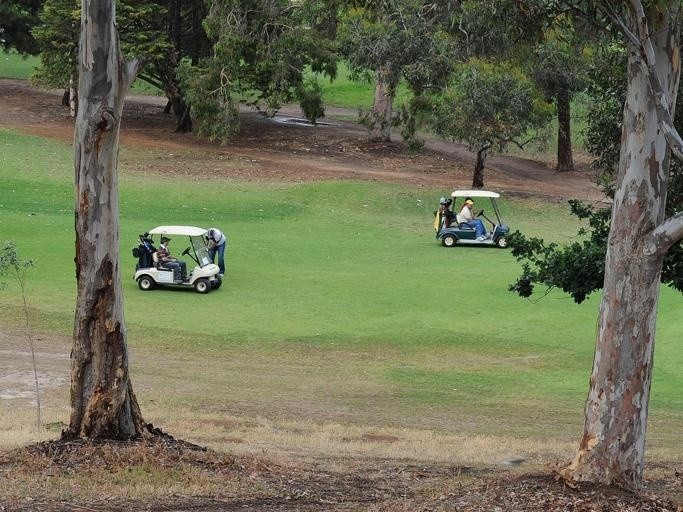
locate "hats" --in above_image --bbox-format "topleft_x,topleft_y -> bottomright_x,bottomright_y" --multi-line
160,237 -> 171,244
207,229 -> 214,238
465,199 -> 475,205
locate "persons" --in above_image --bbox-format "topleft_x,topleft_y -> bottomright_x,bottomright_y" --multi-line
460,200 -> 486,241
157,236 -> 188,285
204,227 -> 226,277
460,197 -> 490,239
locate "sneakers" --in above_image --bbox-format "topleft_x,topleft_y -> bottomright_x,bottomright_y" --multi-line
476,233 -> 489,240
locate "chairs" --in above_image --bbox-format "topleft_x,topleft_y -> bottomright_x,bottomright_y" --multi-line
456,213 -> 475,232
153,252 -> 176,271
